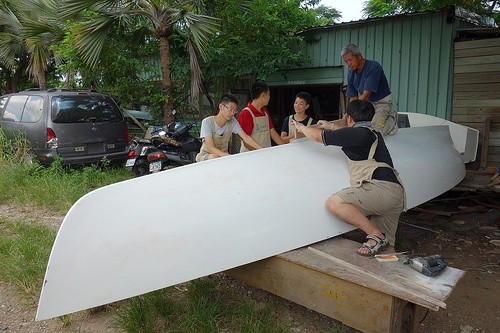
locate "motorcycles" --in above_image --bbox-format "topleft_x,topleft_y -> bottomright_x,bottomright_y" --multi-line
132,119 -> 201,175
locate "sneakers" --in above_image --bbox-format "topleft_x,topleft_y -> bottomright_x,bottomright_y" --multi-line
388,112 -> 399,137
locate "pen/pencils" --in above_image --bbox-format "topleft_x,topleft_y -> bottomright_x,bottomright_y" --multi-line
377,256 -> 395,259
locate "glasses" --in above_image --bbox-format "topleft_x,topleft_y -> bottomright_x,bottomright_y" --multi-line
221,102 -> 239,115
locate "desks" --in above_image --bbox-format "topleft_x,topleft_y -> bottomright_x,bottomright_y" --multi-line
221,237 -> 465,333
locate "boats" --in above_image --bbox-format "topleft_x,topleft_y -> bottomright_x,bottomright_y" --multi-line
34,124 -> 467,321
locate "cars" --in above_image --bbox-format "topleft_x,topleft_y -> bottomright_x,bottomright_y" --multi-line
1,89 -> 130,168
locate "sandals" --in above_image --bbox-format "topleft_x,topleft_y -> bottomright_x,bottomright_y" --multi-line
358,234 -> 390,255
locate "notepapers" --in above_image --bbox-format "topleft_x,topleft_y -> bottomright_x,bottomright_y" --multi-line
375,254 -> 398,262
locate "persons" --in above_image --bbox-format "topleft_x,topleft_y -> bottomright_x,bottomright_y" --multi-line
237,81 -> 283,155
340,43 -> 400,136
194,93 -> 263,163
290,99 -> 407,257
281,91 -> 317,145
